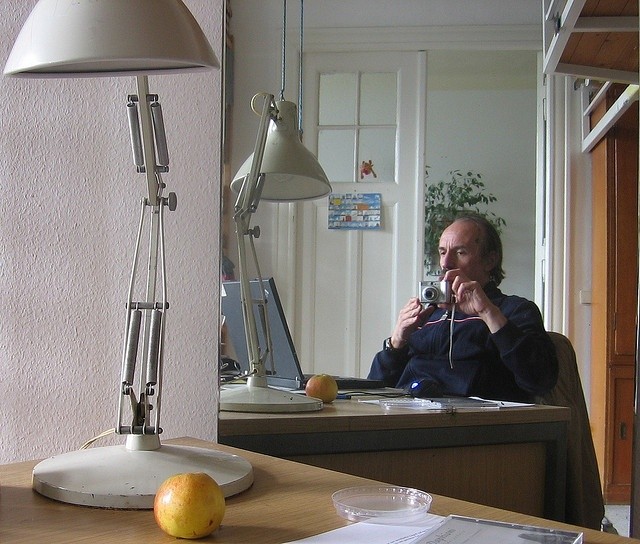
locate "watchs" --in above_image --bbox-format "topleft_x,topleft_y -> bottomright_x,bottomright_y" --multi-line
385,337 -> 407,357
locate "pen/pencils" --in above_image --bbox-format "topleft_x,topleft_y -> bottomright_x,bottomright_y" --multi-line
336,395 -> 352,400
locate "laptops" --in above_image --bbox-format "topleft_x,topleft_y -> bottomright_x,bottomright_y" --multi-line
221,278 -> 385,391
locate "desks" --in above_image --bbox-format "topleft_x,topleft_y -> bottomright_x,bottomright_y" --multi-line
218,405 -> 574,515
1,435 -> 640,543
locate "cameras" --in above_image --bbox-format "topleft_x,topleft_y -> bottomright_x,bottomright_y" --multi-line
418,273 -> 455,305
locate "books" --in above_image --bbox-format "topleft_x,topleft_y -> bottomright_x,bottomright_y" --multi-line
409,514 -> 583,544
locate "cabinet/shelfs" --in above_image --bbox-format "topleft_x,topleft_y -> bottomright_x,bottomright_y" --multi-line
540,0 -> 639,504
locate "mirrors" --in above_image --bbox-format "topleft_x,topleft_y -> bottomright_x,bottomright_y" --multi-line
222,1 -> 638,536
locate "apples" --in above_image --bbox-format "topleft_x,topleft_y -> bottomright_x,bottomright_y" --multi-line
306,373 -> 338,403
155,473 -> 228,539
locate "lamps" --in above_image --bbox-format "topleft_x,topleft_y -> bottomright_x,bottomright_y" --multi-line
230,93 -> 324,412
4,1 -> 253,511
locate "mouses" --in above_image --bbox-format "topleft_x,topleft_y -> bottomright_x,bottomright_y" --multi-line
405,377 -> 444,397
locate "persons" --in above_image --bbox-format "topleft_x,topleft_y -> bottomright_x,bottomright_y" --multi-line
366,213 -> 559,405
